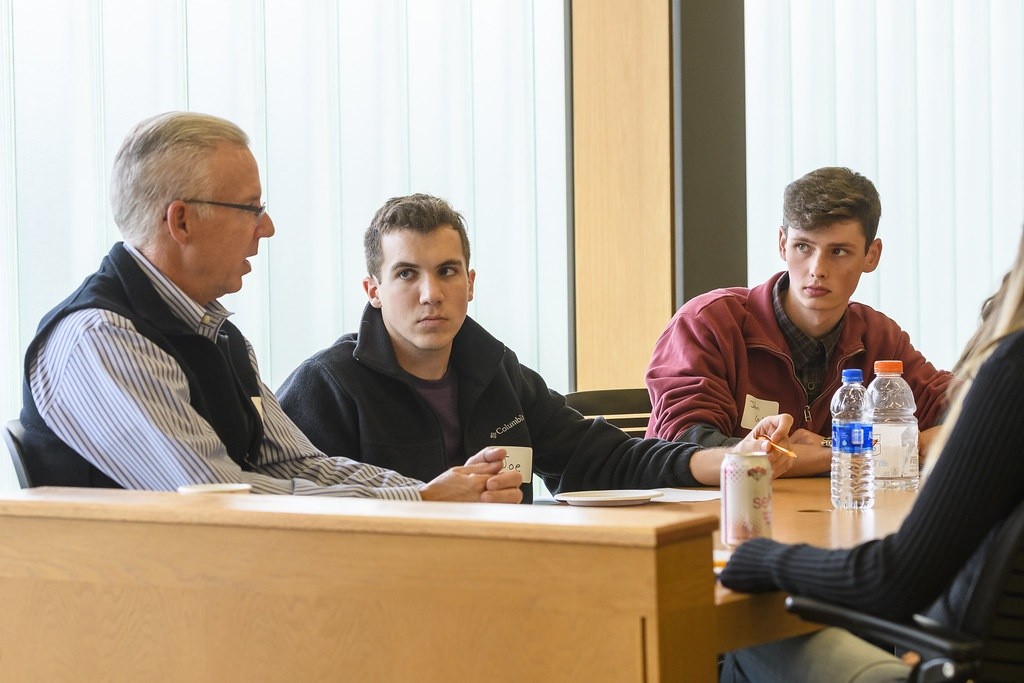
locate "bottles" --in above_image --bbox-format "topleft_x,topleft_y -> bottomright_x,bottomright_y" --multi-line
831,370 -> 875,509
866,361 -> 918,491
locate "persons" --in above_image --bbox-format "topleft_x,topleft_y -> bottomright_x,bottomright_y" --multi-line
270,192 -> 795,507
644,167 -> 954,477
19,111 -> 523,504
719,224 -> 1024,683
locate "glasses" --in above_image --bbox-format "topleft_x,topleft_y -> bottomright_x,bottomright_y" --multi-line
162,197 -> 269,222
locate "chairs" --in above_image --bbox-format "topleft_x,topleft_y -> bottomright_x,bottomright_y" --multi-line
783,500 -> 1024,683
566,389 -> 653,440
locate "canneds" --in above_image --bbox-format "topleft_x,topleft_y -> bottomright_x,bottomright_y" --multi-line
720,451 -> 772,549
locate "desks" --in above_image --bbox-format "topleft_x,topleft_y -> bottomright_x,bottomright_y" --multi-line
0,471 -> 922,683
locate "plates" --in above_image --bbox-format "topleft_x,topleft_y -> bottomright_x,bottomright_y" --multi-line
554,490 -> 663,507
